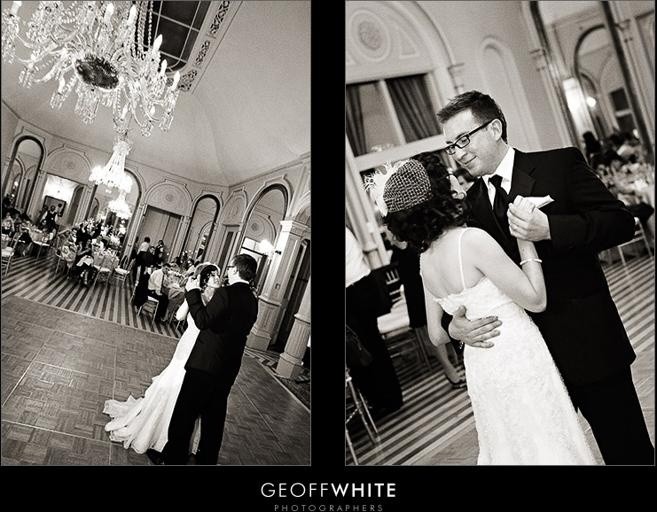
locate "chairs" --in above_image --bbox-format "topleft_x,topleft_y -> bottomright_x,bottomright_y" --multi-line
1,224 -> 188,330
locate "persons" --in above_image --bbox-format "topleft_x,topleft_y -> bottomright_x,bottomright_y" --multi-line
390,240 -> 465,388
438,90 -> 654,464
2,204 -> 104,288
133,236 -> 202,327
147,254 -> 259,466
346,230 -> 404,417
102,262 -> 260,454
382,153 -> 607,466
581,133 -> 635,172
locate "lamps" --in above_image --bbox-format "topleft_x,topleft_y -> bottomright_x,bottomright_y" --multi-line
88,117 -> 133,193
1,0 -> 181,137
108,191 -> 133,220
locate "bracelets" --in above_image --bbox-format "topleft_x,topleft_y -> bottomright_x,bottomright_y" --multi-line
520,258 -> 541,267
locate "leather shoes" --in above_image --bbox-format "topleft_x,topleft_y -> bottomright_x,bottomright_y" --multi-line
146,448 -> 163,465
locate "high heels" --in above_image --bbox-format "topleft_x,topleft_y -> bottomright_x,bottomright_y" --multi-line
444,372 -> 467,388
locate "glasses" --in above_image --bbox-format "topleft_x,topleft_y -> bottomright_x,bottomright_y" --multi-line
443,118 -> 494,156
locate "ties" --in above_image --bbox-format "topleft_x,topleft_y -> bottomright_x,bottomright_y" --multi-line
485,174 -> 512,236
